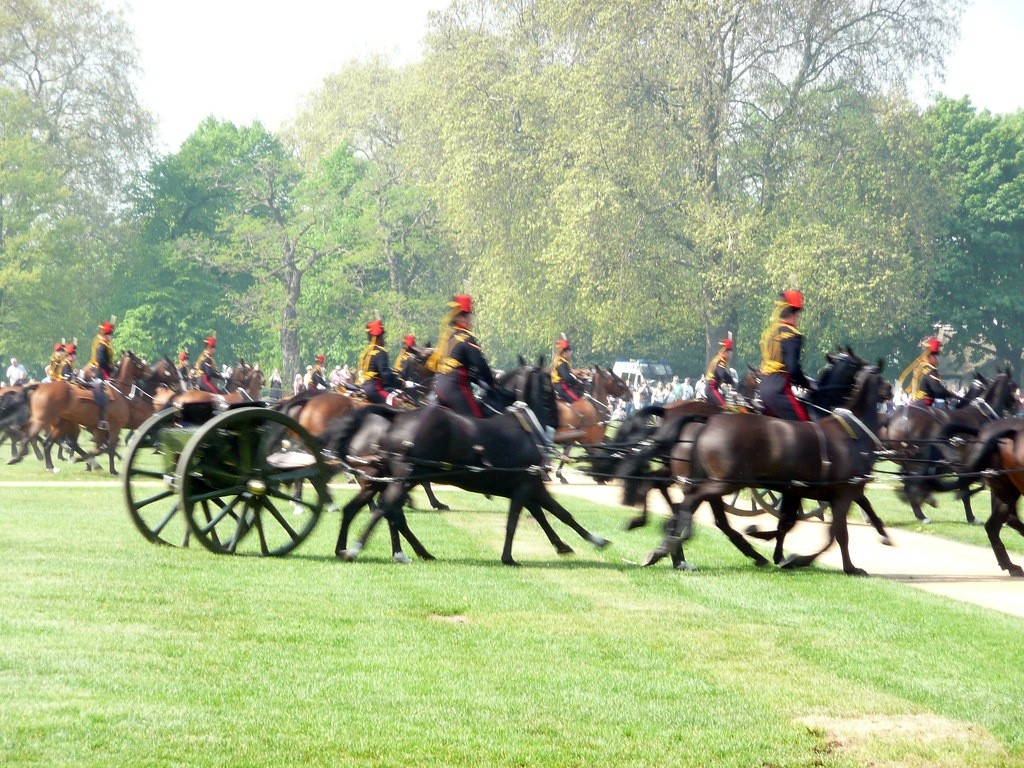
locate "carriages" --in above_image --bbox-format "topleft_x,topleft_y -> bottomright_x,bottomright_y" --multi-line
118,344 -> 1024,579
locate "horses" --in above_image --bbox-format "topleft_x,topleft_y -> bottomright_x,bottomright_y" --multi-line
0,340 -> 1024,578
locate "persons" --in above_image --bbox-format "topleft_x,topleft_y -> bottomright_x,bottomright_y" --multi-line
425,292 -> 516,419
899,338 -> 966,438
360,319 -> 415,405
760,289 -> 875,483
0,322 -> 1023,449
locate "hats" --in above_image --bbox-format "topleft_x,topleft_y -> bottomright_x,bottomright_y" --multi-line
921,338 -> 941,354
98,321 -> 115,337
11,358 -> 17,363
365,320 -> 386,336
54,344 -> 66,352
555,339 -> 569,350
774,291 -> 804,310
447,294 -> 473,316
335,365 -> 341,370
718,339 -> 733,352
66,344 -> 78,356
295,373 -> 302,380
203,338 -> 215,346
314,356 -> 325,364
305,365 -> 312,371
404,336 -> 416,348
